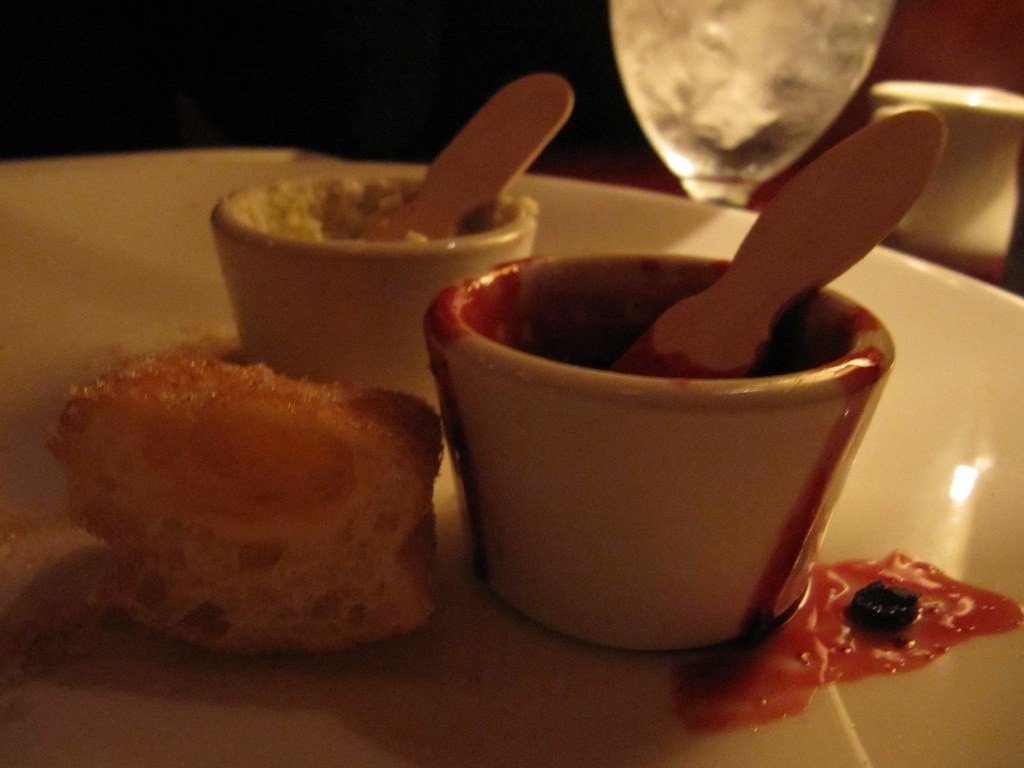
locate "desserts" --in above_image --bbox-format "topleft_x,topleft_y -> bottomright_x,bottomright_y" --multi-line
52,354 -> 444,655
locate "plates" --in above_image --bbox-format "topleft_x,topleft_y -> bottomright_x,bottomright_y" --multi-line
0,152 -> 1023,768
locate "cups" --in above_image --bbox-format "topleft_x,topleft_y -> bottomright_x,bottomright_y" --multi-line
428,254 -> 893,649
213,171 -> 537,412
868,79 -> 1024,284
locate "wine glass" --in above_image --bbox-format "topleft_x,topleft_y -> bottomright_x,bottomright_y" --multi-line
611,0 -> 895,208
